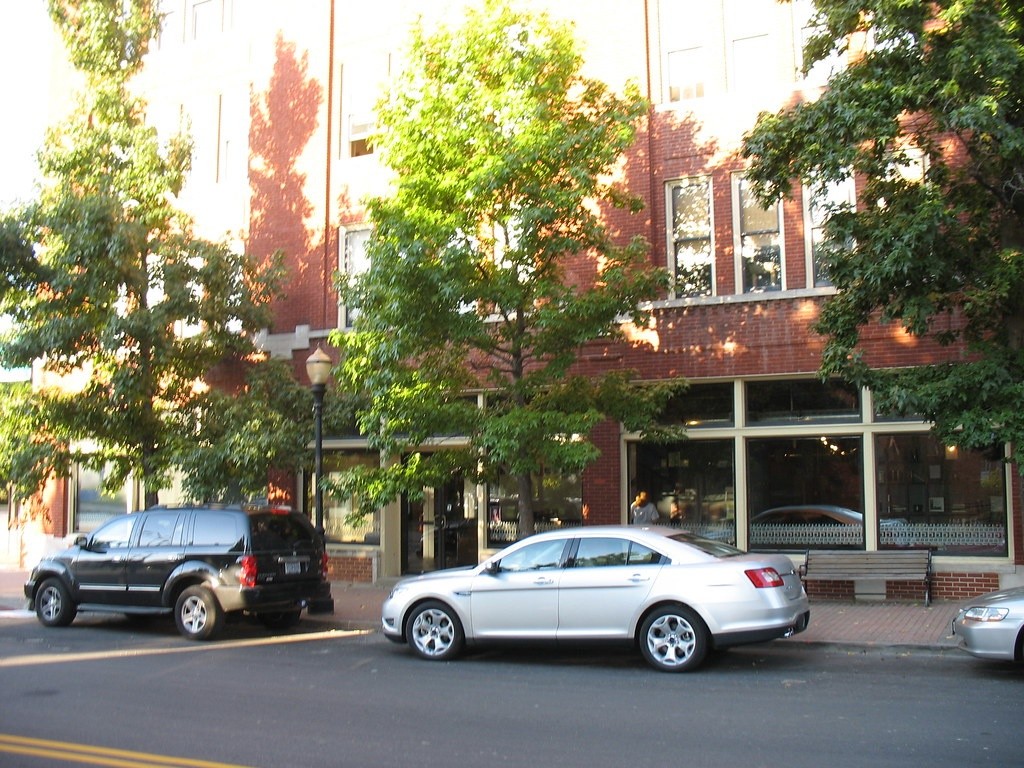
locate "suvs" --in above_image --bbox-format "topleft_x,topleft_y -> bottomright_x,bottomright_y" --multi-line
23,505 -> 329,641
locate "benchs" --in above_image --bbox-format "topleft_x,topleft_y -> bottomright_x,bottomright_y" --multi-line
799,547 -> 933,608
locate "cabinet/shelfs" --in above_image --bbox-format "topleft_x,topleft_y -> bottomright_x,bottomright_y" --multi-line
877,434 -> 950,523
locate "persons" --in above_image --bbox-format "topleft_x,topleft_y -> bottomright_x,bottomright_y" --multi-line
630,489 -> 659,526
670,499 -> 685,525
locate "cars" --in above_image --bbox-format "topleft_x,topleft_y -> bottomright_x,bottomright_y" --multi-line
747,503 -> 909,552
951,582 -> 1024,677
381,525 -> 810,673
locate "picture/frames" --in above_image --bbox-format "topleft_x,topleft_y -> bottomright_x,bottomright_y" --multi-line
929,497 -> 945,512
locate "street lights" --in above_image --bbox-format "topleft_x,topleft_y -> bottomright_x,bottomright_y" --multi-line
304,343 -> 335,614
